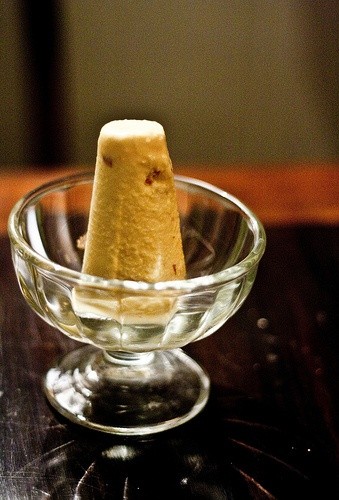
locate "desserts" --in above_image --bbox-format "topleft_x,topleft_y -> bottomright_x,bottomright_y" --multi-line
75,118 -> 186,316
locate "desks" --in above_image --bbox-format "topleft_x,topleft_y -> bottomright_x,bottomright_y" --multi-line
0,218 -> 338,500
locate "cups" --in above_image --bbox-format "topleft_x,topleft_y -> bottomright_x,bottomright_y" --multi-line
6,170 -> 268,438
